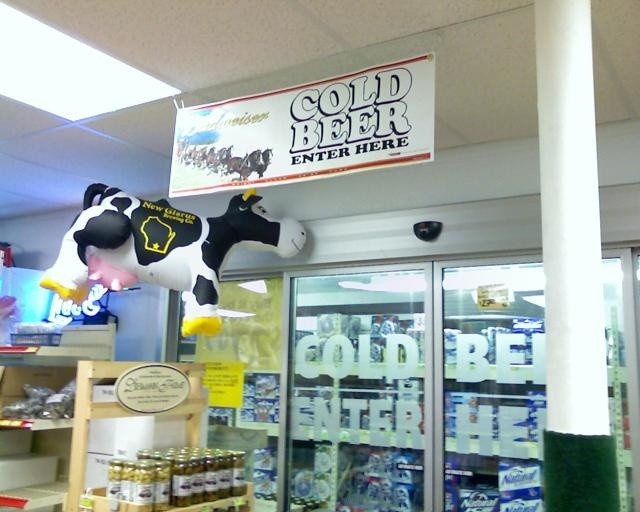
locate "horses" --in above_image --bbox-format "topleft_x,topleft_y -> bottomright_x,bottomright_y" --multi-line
181,145 -> 274,181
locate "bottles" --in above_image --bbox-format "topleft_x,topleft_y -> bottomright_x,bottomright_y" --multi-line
232,451 -> 246,495
122,461 -> 136,500
174,458 -> 190,507
136,463 -> 154,502
190,456 -> 203,503
204,454 -> 218,501
107,459 -> 121,496
219,453 -> 231,498
153,460 -> 170,510
138,446 -> 227,461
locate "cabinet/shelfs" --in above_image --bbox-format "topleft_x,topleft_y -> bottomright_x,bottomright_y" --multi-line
159,243 -> 640,512
66,363 -> 251,512
0,312 -> 115,510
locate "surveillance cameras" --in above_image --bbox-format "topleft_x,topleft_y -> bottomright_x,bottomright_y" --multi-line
413,220 -> 443,242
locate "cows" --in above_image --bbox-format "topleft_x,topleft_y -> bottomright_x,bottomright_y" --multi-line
38,182 -> 306,338
